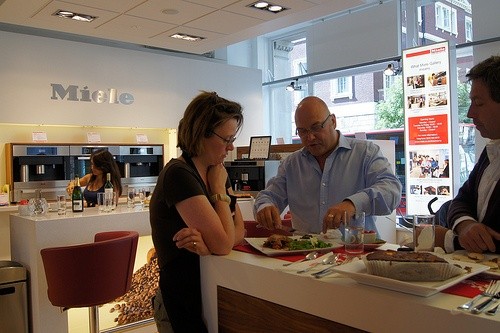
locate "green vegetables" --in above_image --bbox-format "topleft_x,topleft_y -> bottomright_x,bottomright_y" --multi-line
288,237 -> 333,250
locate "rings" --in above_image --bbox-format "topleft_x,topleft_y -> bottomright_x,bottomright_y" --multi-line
194,242 -> 196,247
328,215 -> 333,218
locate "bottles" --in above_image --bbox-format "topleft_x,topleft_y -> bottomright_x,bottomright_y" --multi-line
235,180 -> 240,191
72,175 -> 83,212
26,189 -> 49,216
104,173 -> 113,199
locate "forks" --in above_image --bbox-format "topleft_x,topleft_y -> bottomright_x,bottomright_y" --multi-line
457,279 -> 500,311
311,256 -> 354,276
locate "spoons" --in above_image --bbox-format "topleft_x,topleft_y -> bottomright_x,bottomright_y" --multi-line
283,252 -> 316,266
297,254 -> 337,274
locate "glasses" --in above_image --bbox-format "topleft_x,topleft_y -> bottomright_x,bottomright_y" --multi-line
296,114 -> 329,135
211,132 -> 236,146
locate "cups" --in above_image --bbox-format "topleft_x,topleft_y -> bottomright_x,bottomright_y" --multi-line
345,210 -> 365,254
17,186 -> 153,216
413,215 -> 435,252
244,186 -> 251,189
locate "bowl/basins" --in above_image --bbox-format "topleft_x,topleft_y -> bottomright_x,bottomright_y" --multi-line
363,232 -> 377,244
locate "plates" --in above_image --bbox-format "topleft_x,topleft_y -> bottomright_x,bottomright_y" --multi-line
445,250 -> 500,279
243,234 -> 344,257
337,238 -> 386,249
241,189 -> 253,191
332,258 -> 490,298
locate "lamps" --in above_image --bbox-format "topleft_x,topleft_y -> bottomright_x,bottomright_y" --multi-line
385,59 -> 403,76
286,78 -> 302,91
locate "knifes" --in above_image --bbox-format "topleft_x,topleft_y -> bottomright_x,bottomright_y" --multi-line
471,291 -> 500,315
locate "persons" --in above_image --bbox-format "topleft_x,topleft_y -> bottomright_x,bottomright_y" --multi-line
67,149 -> 122,207
413,55 -> 500,253
415,77 -> 421,86
254,96 -> 402,243
420,97 -> 424,107
425,187 -> 435,194
420,155 -> 449,177
149,92 -> 246,332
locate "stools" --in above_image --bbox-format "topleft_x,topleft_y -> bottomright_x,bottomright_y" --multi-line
40,231 -> 139,333
243,212 -> 293,238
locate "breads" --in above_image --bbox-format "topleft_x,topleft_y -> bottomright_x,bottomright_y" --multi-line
366,250 -> 471,273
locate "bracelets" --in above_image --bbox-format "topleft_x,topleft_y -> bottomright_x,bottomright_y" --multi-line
211,194 -> 231,205
